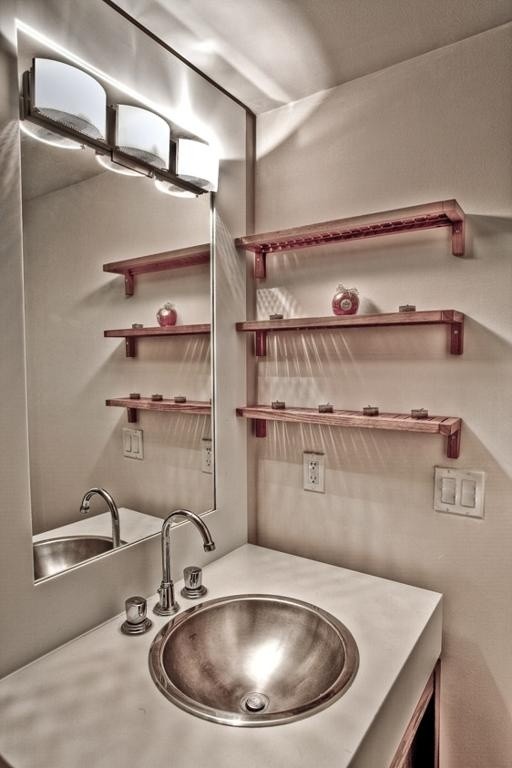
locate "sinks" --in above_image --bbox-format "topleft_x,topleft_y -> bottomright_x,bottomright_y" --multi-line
32,533 -> 129,582
141,590 -> 360,728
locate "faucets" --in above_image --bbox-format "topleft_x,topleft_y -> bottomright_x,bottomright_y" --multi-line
78,485 -> 125,549
152,507 -> 216,617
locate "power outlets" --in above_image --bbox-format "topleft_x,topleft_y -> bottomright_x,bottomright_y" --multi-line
201,436 -> 214,473
301,450 -> 326,495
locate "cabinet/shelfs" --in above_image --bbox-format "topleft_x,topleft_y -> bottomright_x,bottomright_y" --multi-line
98,241 -> 213,426
232,198 -> 471,459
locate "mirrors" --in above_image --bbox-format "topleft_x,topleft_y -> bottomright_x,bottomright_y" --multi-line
6,15 -> 221,586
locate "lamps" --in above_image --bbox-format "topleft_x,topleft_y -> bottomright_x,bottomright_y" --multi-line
29,54 -> 220,194
